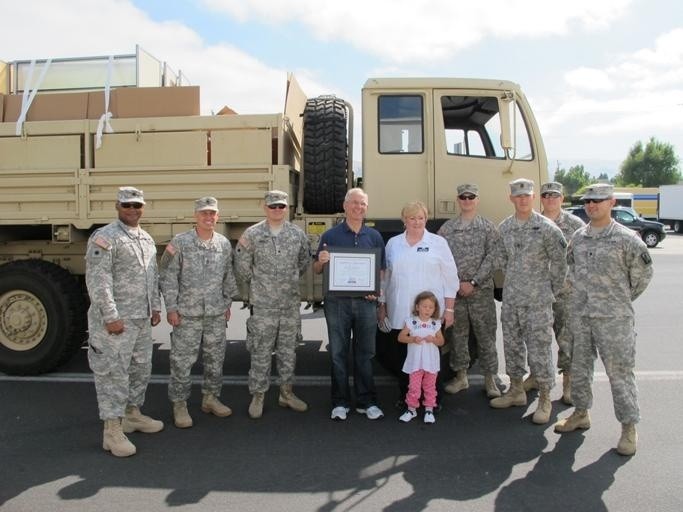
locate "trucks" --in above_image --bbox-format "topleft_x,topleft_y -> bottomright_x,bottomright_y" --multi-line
571,185 -> 682,232
0,77 -> 551,384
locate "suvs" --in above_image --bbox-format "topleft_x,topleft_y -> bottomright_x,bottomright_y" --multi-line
561,206 -> 669,250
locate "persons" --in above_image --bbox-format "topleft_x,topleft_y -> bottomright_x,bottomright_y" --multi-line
490,179 -> 569,426
83,185 -> 164,457
314,186 -> 385,421
397,291 -> 445,424
436,183 -> 501,399
378,200 -> 460,415
160,195 -> 237,428
523,182 -> 587,405
234,189 -> 314,419
554,182 -> 654,456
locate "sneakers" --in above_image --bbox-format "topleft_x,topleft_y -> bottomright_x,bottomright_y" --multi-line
424,411 -> 436,424
330,405 -> 351,421
398,410 -> 418,423
355,406 -> 385,420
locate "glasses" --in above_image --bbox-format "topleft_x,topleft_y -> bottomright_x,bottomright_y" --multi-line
265,203 -> 287,209
541,192 -> 562,199
458,194 -> 478,200
118,201 -> 143,210
583,199 -> 610,204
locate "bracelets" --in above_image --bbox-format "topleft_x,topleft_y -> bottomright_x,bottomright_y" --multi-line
444,309 -> 455,313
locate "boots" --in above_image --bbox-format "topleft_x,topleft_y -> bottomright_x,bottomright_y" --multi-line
102,404 -> 165,458
247,391 -> 265,418
616,421 -> 639,456
554,408 -> 591,433
488,377 -> 527,408
561,372 -> 575,406
200,392 -> 233,417
444,368 -> 469,394
532,388 -> 553,424
523,374 -> 539,392
278,382 -> 309,413
171,399 -> 193,428
484,371 -> 502,398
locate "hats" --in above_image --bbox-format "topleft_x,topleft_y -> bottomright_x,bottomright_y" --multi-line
539,182 -> 562,196
509,177 -> 535,196
456,184 -> 480,196
263,190 -> 288,207
579,184 -> 614,201
115,186 -> 149,207
194,196 -> 219,215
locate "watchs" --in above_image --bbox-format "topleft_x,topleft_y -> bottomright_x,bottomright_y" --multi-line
469,279 -> 479,287
